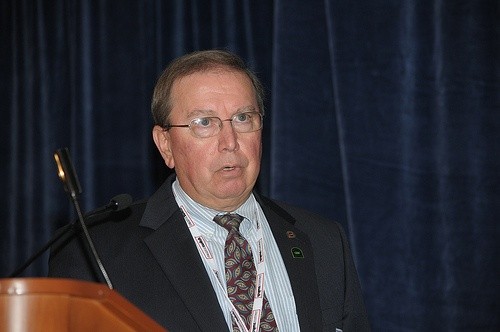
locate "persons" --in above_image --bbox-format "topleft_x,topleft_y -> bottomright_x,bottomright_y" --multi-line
48,50 -> 373,332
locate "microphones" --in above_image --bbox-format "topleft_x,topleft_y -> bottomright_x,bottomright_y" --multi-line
6,193 -> 136,277
55,144 -> 117,290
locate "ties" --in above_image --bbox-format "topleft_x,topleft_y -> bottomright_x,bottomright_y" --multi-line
213,214 -> 279,331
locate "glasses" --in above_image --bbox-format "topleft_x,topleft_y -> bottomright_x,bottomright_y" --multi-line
164,111 -> 265,137
